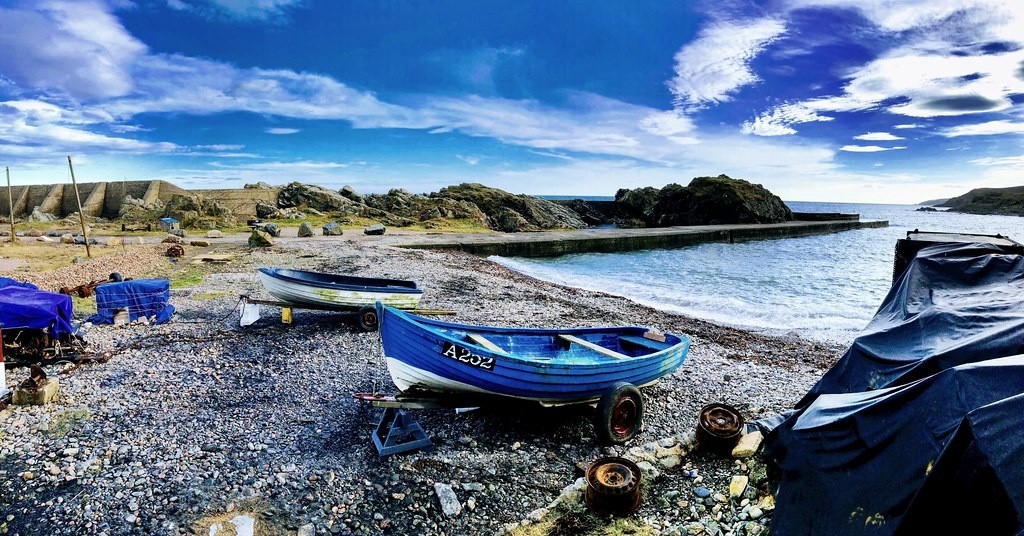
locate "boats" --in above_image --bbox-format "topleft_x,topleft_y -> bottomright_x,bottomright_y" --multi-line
256,267 -> 424,310
756,228 -> 1023,536
375,301 -> 691,402
